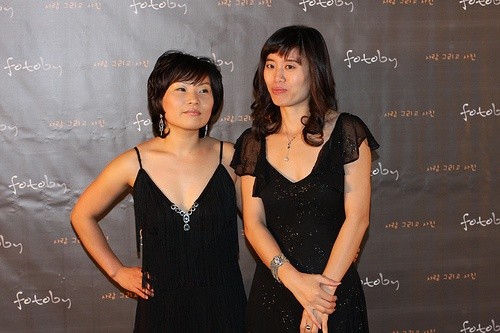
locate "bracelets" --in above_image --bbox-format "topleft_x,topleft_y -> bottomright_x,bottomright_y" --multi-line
269,252 -> 288,284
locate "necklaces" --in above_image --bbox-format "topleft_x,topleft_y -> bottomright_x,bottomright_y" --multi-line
280,123 -> 306,162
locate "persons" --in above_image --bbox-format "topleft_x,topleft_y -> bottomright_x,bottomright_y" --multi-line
69,48 -> 360,333
226,24 -> 379,333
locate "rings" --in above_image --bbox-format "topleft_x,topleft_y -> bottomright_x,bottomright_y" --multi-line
305,325 -> 311,330
